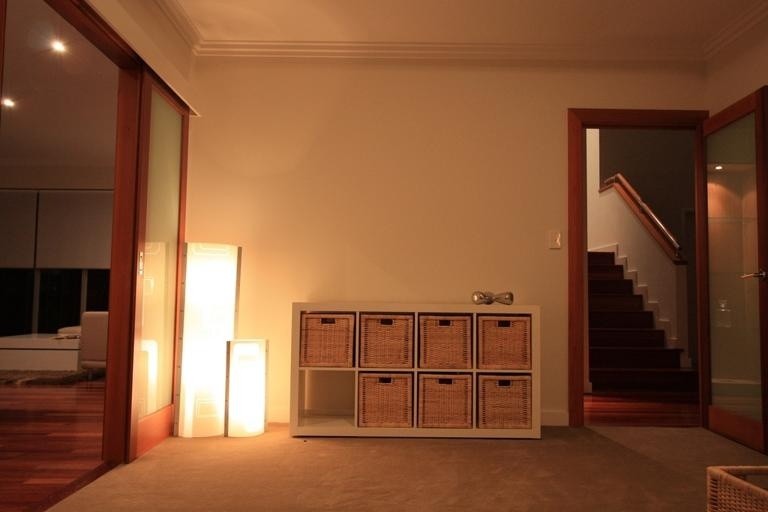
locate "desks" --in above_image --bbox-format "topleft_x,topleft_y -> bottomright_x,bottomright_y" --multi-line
0,334 -> 79,371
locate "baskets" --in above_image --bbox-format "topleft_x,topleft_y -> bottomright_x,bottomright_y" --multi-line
357,315 -> 532,429
706,464 -> 767,512
300,314 -> 354,368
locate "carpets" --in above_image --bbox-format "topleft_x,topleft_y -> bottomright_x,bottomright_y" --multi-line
43,420 -> 768,512
0,369 -> 105,387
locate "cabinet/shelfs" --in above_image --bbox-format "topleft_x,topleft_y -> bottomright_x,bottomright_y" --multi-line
290,301 -> 541,441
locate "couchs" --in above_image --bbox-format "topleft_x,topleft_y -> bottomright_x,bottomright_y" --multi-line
58,312 -> 108,369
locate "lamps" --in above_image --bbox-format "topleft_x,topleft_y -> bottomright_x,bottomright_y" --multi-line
172,242 -> 242,437
224,340 -> 267,438
